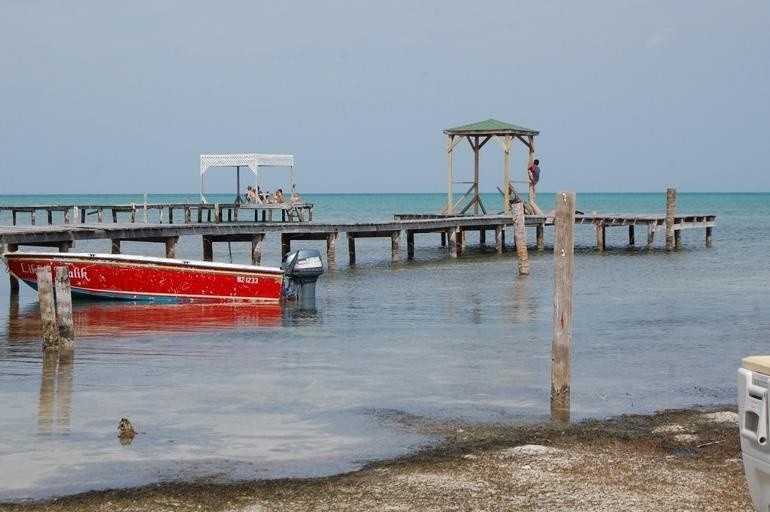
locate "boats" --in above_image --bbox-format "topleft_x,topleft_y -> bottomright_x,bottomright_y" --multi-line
3,248 -> 324,316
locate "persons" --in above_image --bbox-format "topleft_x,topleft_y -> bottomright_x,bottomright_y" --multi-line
247,184 -> 301,204
528,159 -> 541,197
